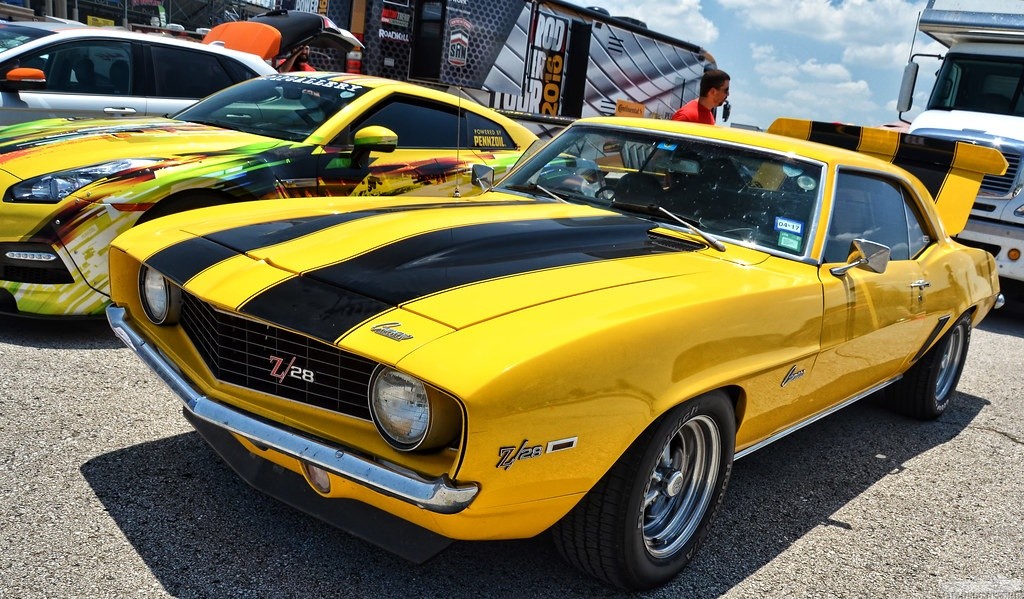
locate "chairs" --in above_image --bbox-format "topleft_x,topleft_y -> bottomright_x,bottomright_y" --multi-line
968,92 -> 1012,115
614,171 -> 665,206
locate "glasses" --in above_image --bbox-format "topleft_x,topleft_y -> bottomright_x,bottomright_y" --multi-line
717,88 -> 729,94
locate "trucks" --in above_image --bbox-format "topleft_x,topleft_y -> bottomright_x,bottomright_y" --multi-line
891,0 -> 1024,302
259,1 -> 730,129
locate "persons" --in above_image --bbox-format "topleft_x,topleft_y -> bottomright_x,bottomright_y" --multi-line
278,45 -> 316,74
652,69 -> 731,189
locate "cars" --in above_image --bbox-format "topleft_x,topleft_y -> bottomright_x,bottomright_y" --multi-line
105,117 -> 1007,591
0,12 -> 368,191
0,72 -> 541,338
